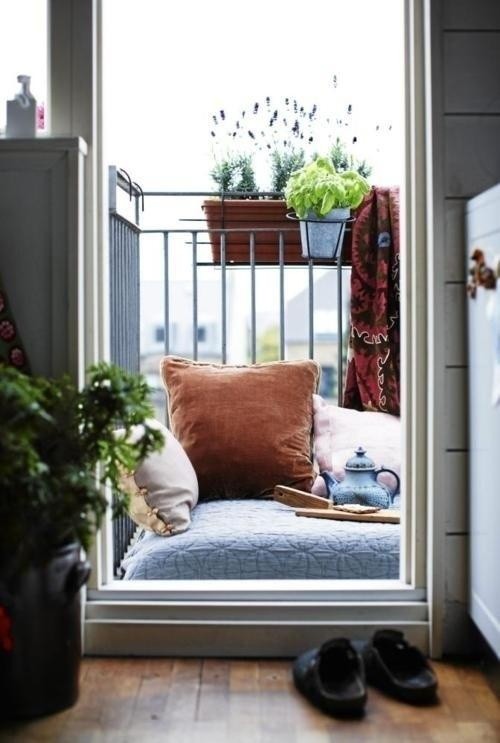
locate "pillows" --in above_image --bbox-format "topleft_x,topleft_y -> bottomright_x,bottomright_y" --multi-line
306,390 -> 402,499
158,351 -> 319,501
101,424 -> 201,537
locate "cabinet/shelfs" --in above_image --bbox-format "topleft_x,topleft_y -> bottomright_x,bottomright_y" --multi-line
2,137 -> 92,391
465,183 -> 500,667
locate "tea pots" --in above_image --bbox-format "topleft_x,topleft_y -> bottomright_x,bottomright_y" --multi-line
319,445 -> 401,509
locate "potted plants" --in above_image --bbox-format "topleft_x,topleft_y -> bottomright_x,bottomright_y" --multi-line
203,93 -> 392,265
284,155 -> 370,257
0,355 -> 150,726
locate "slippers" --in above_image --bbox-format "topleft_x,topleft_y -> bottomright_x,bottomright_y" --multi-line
293,638 -> 366,714
352,629 -> 437,701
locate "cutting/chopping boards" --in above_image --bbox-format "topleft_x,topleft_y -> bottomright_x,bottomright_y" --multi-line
272,484 -> 400,525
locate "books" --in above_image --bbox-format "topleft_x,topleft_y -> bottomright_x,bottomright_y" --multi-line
294,503 -> 400,526
